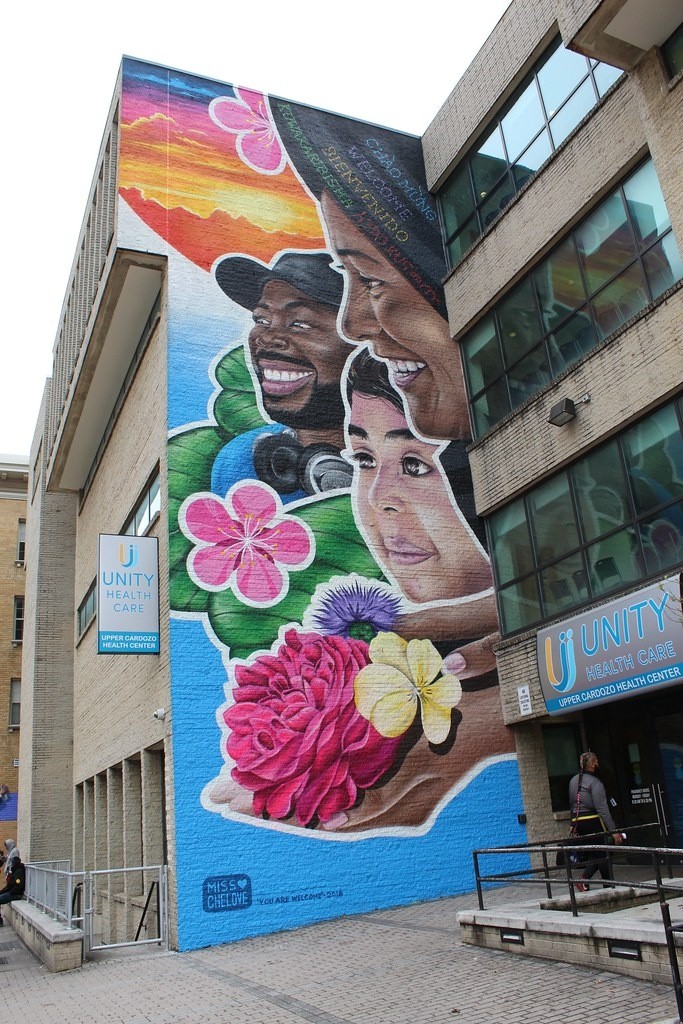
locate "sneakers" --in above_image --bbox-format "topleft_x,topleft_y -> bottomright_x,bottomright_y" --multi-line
575,878 -> 590,892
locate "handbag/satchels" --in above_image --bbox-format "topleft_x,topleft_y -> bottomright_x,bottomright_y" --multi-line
557,842 -> 585,867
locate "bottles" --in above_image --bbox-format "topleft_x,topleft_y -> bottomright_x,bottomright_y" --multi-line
607,833 -> 627,842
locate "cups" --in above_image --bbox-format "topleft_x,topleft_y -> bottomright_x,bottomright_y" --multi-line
6,871 -> 12,880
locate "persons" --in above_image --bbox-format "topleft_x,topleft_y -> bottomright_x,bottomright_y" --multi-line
0,856 -> 25,927
0,838 -> 19,883
569,752 -> 622,891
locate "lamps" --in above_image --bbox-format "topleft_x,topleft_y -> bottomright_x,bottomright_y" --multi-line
547,398 -> 583,428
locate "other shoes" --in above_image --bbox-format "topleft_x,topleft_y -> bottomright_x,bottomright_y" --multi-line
0,917 -> 4,927
